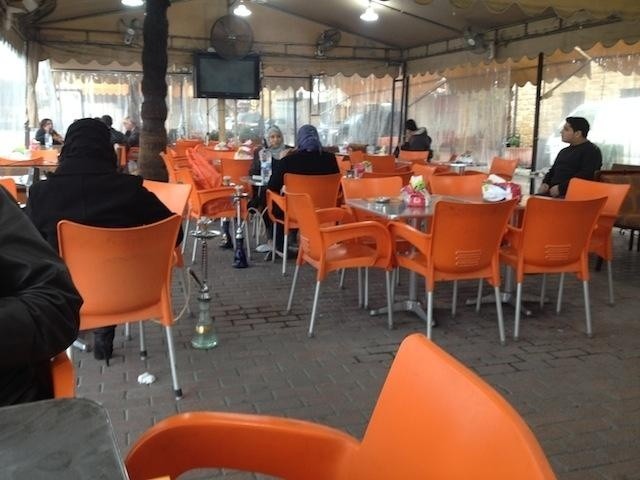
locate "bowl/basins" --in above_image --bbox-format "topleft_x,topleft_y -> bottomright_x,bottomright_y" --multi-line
449,163 -> 467,177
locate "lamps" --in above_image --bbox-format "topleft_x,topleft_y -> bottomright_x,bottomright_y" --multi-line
233,0 -> 252,16
360,0 -> 379,21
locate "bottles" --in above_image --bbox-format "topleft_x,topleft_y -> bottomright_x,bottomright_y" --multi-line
258,148 -> 274,186
204,132 -> 211,147
43,127 -> 54,150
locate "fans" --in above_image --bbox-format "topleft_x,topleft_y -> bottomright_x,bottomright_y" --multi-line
461,23 -> 498,60
210,14 -> 254,61
314,29 -> 342,59
116,14 -> 143,45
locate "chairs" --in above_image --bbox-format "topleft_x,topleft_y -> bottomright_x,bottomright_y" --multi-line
500,196 -> 606,340
390,198 -> 520,345
124,332 -> 555,478
1,135 -> 639,260
566,176 -> 630,306
124,180 -> 194,341
56,213 -> 184,401
283,190 -> 396,336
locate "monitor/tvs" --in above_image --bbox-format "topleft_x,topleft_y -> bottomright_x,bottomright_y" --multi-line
193,51 -> 260,99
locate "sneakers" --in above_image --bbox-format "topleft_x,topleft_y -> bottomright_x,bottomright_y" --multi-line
257,244 -> 272,252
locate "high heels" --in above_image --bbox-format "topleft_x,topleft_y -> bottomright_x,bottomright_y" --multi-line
94,334 -> 113,365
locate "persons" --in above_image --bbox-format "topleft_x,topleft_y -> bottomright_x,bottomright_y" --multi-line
405,120 -> 429,161
36,119 -> 65,178
247,126 -> 297,246
25,118 -> 183,360
0,186 -> 84,407
123,116 -> 139,151
538,117 -> 602,199
100,115 -> 129,152
256,125 -> 340,258
